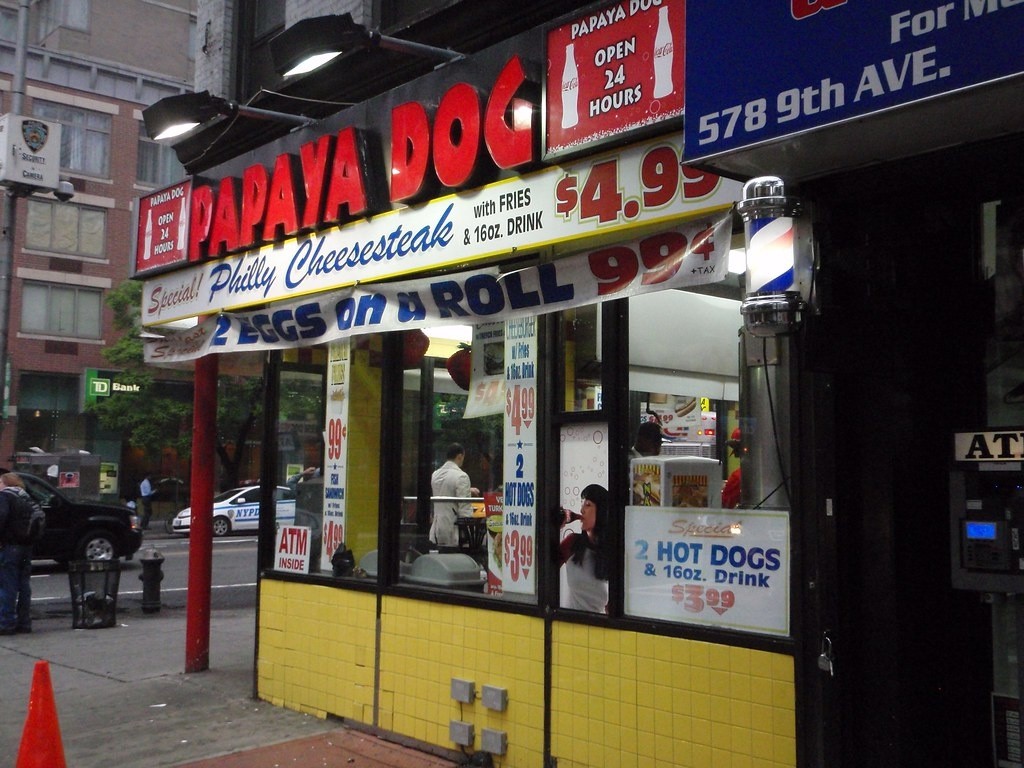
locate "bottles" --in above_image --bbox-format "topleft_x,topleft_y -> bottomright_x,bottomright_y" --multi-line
561,509 -> 583,525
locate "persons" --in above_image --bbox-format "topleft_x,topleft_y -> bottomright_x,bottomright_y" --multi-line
558,485 -> 609,614
721,429 -> 743,509
0,473 -> 34,634
629,421 -> 662,462
126,472 -> 158,528
429,442 -> 481,554
287,467 -> 324,495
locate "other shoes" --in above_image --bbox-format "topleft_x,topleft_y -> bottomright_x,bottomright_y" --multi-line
142,527 -> 151,530
0,627 -> 14,635
14,624 -> 32,634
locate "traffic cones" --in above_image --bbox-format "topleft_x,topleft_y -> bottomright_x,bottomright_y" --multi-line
15,660 -> 67,766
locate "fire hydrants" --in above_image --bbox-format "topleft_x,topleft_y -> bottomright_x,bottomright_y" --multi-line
136,543 -> 168,614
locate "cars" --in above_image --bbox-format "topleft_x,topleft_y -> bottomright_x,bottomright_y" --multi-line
173,484 -> 297,538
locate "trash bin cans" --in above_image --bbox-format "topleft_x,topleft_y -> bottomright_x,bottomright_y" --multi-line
402,551 -> 486,594
66,557 -> 123,630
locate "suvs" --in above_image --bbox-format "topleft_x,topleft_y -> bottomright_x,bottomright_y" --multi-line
15,471 -> 146,572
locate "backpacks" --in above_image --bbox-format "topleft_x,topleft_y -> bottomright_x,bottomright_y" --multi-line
3,485 -> 46,543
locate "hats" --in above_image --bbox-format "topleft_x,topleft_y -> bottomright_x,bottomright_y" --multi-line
725,428 -> 742,447
